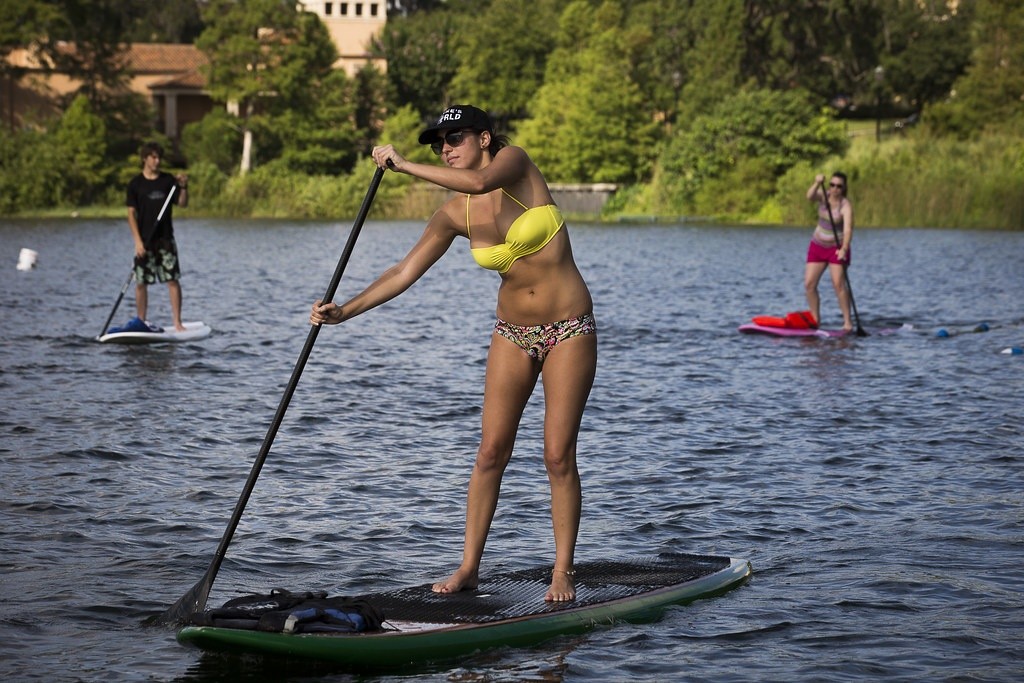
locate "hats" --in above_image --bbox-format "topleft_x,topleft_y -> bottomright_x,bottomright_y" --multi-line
418,104 -> 492,144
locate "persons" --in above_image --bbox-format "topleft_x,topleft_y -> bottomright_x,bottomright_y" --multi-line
307,105 -> 598,602
124,141 -> 189,333
805,171 -> 854,330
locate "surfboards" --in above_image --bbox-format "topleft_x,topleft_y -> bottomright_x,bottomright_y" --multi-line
738,322 -> 851,337
100,320 -> 213,343
175,551 -> 753,657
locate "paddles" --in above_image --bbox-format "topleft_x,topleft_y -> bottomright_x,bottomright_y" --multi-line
820,177 -> 869,335
96,178 -> 179,339
166,147 -> 393,614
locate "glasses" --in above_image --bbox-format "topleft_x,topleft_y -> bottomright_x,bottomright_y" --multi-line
830,183 -> 844,188
430,129 -> 475,155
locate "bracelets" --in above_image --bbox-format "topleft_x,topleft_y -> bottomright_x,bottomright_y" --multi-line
180,185 -> 187,190
